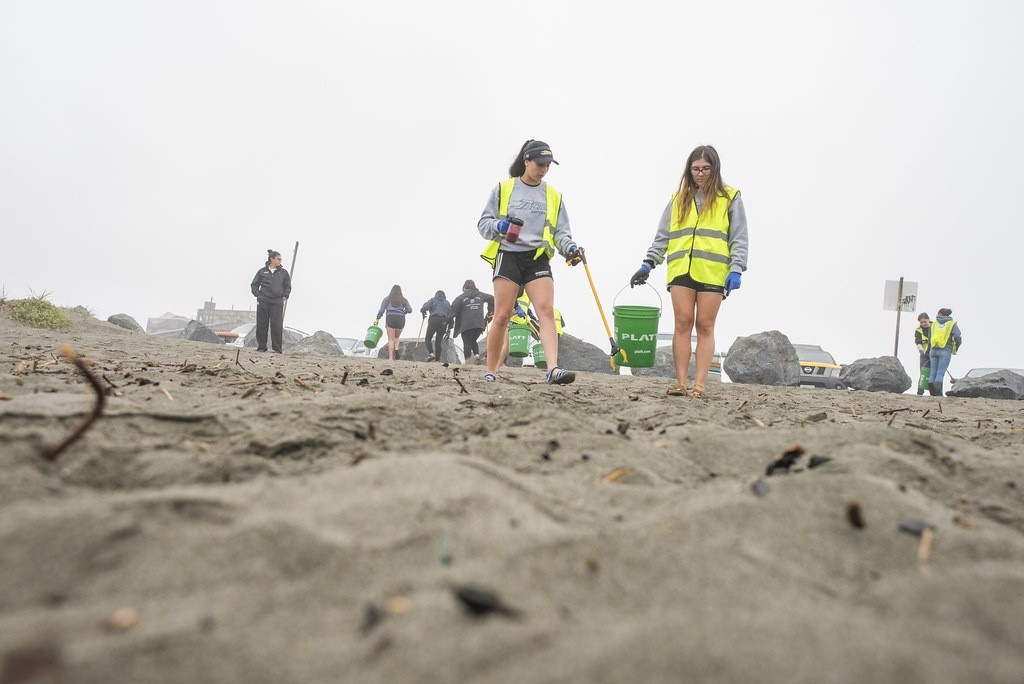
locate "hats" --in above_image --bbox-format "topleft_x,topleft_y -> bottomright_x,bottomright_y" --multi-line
523,146 -> 559,166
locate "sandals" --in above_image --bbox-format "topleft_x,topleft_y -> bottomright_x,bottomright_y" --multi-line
545,365 -> 575,384
483,373 -> 496,382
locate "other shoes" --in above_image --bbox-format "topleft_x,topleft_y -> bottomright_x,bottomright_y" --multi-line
667,385 -> 688,396
929,382 -> 943,396
425,354 -> 436,362
395,350 -> 400,360
474,355 -> 480,365
689,389 -> 704,397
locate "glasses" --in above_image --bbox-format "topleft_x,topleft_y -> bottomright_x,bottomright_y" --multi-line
690,168 -> 712,176
273,258 -> 282,261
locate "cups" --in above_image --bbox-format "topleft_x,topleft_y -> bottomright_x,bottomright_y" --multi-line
505,218 -> 524,243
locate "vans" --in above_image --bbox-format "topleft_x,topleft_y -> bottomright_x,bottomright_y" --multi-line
224,324 -> 311,351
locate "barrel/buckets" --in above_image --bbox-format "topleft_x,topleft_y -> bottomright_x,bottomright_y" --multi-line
612,280 -> 662,367
364,324 -> 383,348
919,360 -> 930,390
533,344 -> 546,366
508,315 -> 532,357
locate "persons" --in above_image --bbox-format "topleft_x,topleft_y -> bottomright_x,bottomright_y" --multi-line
421,291 -> 454,363
915,312 -> 933,395
928,308 -> 962,396
372,285 -> 413,360
448,280 -> 494,365
476,139 -> 581,383
502,285 -> 539,367
553,307 -> 566,339
251,249 -> 291,353
630,144 -> 749,399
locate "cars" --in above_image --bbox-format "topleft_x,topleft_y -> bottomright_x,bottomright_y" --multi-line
949,367 -> 1024,384
334,336 -> 379,358
790,343 -> 845,388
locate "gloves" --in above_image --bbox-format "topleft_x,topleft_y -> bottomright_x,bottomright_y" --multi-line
920,350 -> 928,362
566,247 -> 581,266
498,220 -> 509,235
422,313 -> 427,317
725,272 -> 741,296
630,264 -> 650,288
373,320 -> 379,326
